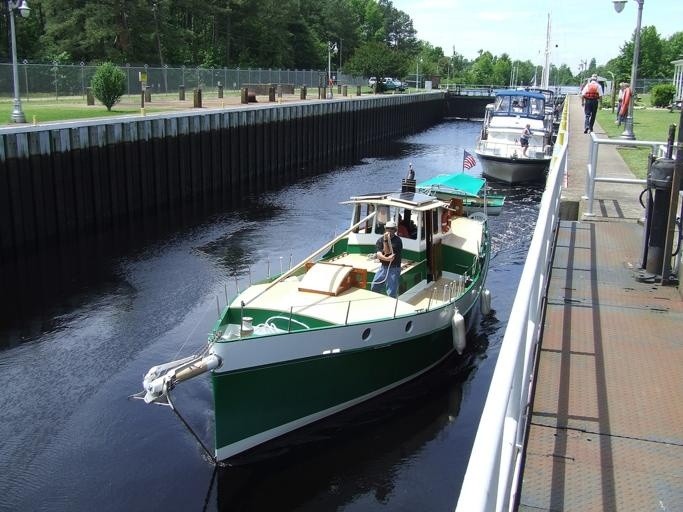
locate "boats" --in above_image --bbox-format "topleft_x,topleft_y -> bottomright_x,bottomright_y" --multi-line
128,172 -> 505,467
475,86 -> 565,184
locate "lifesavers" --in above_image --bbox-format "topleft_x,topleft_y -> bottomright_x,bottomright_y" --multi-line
441,212 -> 451,234
618,87 -> 631,116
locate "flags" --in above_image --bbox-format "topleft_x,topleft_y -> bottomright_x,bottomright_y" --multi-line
463,150 -> 476,170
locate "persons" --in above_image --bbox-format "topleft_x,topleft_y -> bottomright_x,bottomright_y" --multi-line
519,124 -> 533,157
581,77 -> 603,134
369,221 -> 402,299
616,81 -> 624,120
614,83 -> 631,126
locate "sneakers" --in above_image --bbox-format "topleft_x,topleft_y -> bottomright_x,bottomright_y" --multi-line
583,127 -> 593,134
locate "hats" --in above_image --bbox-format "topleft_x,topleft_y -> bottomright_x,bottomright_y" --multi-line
382,221 -> 398,229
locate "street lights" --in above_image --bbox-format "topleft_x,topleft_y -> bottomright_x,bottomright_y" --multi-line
416,55 -> 423,93
613,0 -> 644,147
327,42 -> 338,99
447,63 -> 454,92
8,1 -> 32,122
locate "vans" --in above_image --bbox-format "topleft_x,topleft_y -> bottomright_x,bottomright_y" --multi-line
368,77 -> 407,91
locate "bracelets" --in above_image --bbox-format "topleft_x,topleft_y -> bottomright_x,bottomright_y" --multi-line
383,239 -> 388,242
